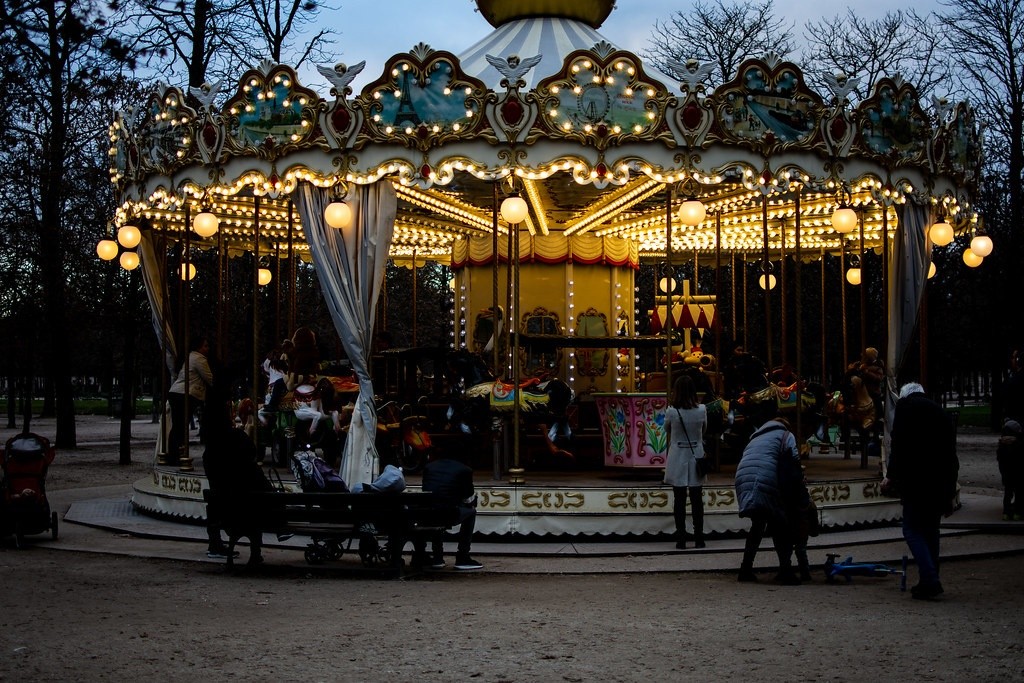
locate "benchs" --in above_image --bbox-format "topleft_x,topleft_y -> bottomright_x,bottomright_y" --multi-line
203,488 -> 454,580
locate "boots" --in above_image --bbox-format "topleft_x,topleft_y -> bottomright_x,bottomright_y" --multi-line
738,563 -> 758,584
778,560 -> 800,585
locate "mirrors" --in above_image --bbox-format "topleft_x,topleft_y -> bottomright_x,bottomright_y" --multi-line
517,305 -> 563,376
573,307 -> 610,376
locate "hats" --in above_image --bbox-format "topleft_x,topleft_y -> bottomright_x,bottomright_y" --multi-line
863,346 -> 878,359
1004,420 -> 1020,432
897,382 -> 925,397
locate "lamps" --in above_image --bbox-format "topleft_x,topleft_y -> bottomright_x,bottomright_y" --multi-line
926,214 -> 993,280
831,180 -> 857,233
500,168 -> 528,224
658,264 -> 677,293
676,173 -> 706,228
259,255 -> 272,286
759,260 -> 776,290
97,192 -> 219,280
323,170 -> 351,228
846,253 -> 861,286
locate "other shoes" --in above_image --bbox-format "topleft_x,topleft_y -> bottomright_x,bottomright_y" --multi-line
1002,514 -> 1009,520
910,584 -> 941,602
675,544 -> 686,548
695,542 -> 705,548
798,576 -> 812,584
1013,513 -> 1021,520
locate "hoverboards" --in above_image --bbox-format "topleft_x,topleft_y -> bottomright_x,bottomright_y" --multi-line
824,553 -> 908,591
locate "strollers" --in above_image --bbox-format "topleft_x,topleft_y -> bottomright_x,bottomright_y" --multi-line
288,448 -> 393,567
0,432 -> 58,550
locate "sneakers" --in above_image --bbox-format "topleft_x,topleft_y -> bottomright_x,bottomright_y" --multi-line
453,551 -> 483,569
207,544 -> 241,558
433,554 -> 446,568
276,528 -> 296,541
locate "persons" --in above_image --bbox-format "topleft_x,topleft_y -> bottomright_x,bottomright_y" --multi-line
845,347 -> 885,436
884,381 -> 958,602
422,446 -> 484,570
996,420 -> 1024,520
664,376 -> 708,549
164,337 -> 214,467
238,338 -> 345,464
200,402 -> 296,558
352,464 -> 409,568
722,342 -> 766,396
734,417 -> 814,582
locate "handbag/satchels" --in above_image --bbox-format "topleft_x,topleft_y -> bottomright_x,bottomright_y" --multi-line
291,445 -> 349,494
694,456 -> 720,477
266,466 -> 292,494
